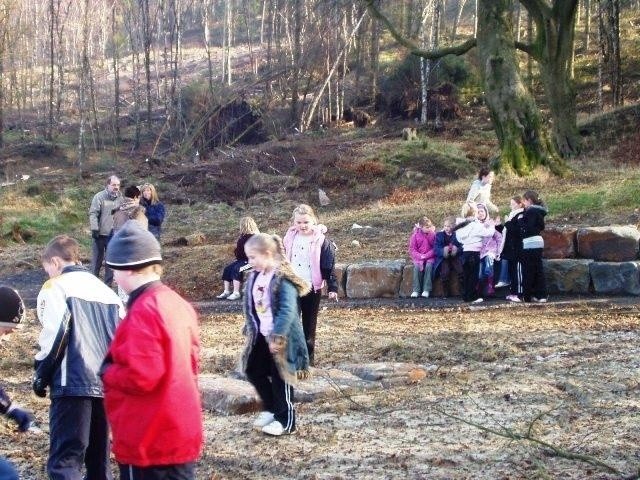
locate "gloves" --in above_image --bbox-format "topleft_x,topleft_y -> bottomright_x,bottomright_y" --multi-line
8,408 -> 33,431
92,231 -> 99,239
33,378 -> 47,397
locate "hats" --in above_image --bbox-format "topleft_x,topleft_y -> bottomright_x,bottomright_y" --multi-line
0,287 -> 24,329
105,219 -> 162,270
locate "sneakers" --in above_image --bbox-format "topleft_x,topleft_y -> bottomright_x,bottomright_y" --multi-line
227,292 -> 241,300
410,279 -> 549,304
254,411 -> 274,426
217,293 -> 230,298
262,421 -> 298,436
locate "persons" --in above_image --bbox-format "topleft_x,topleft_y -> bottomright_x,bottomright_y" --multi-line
89,176 -> 125,287
140,182 -> 167,240
112,186 -> 148,300
215,216 -> 260,301
1,285 -> 36,480
282,204 -> 338,366
101,220 -> 204,480
241,232 -> 312,436
408,168 -> 549,304
33,236 -> 128,480
